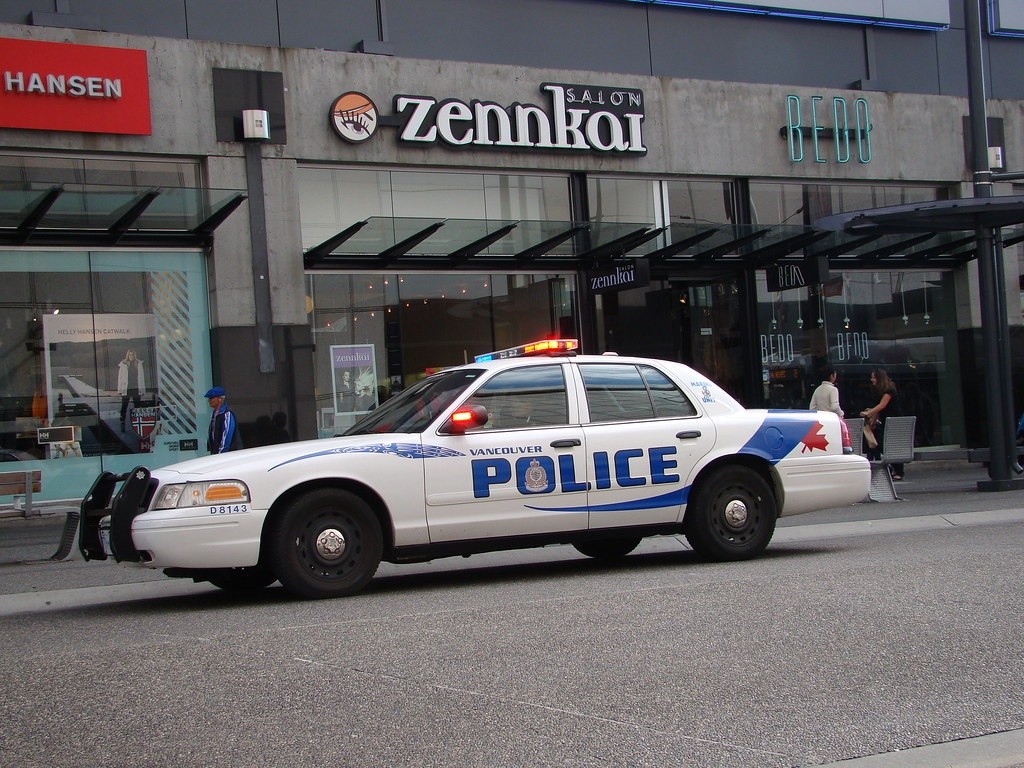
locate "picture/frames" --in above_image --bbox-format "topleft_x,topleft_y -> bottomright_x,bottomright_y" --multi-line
330,343 -> 379,415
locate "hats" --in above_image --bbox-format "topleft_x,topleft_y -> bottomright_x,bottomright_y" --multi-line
204,387 -> 226,398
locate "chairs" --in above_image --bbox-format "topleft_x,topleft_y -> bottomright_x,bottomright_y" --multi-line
844,418 -> 864,456
868,416 -> 916,503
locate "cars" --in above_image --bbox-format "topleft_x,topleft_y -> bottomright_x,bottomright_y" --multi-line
74,337 -> 874,597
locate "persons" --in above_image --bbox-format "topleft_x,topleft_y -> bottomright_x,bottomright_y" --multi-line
810,364 -> 845,421
338,370 -> 361,413
204,386 -> 237,462
860,366 -> 906,482
863,402 -> 885,471
117,346 -> 146,436
